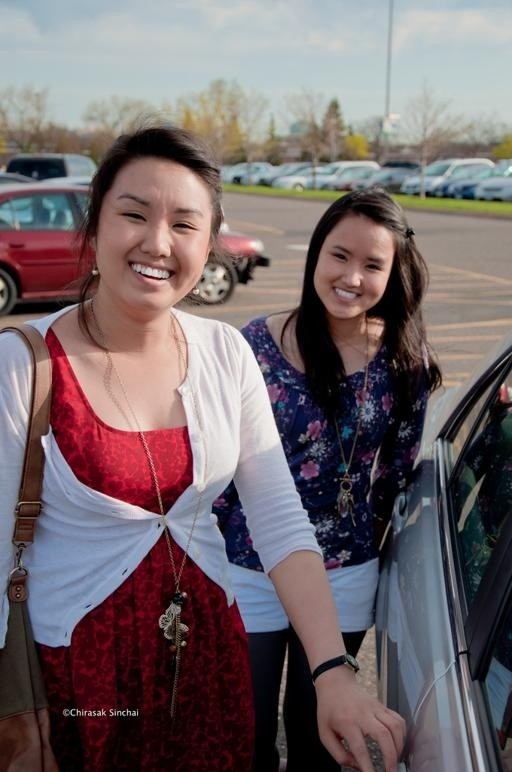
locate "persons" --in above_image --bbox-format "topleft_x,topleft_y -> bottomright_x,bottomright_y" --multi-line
211,184 -> 444,772
0,122 -> 410,772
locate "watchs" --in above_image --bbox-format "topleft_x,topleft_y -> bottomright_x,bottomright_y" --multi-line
310,654 -> 360,686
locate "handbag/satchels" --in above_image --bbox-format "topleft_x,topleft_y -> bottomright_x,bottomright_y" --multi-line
0,568 -> 58,771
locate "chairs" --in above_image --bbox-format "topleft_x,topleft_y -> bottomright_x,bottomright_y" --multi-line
32,207 -> 74,229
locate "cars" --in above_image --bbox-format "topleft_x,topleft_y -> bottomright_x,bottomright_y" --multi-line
0,182 -> 269,316
222,157 -> 511,201
376,332 -> 512,772
1,148 -> 97,185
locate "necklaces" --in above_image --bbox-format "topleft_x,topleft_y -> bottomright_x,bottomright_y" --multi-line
335,317 -> 369,526
88,297 -> 207,721
